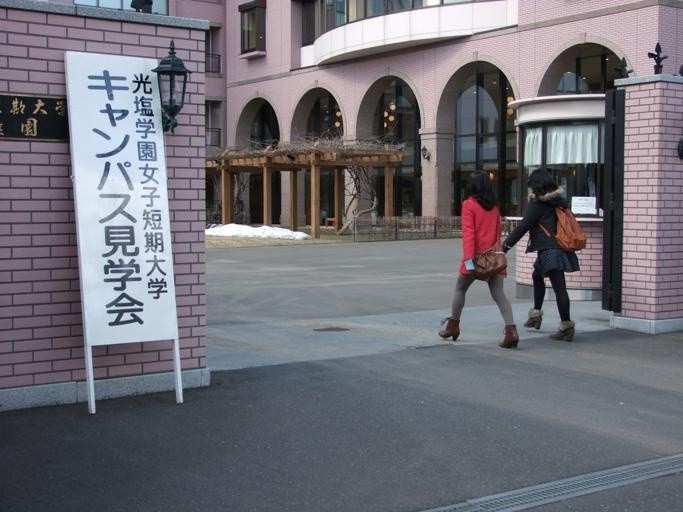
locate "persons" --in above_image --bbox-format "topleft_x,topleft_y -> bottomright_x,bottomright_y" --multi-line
502,168 -> 580,342
678,138 -> 683,161
439,169 -> 519,348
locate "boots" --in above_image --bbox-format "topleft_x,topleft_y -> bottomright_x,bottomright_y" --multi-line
549,320 -> 575,342
524,309 -> 544,329
439,317 -> 461,341
499,324 -> 519,349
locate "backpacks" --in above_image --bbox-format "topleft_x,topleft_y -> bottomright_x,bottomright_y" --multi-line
538,206 -> 587,252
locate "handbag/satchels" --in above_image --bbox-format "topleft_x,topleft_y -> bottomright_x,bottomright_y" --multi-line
472,247 -> 507,281
